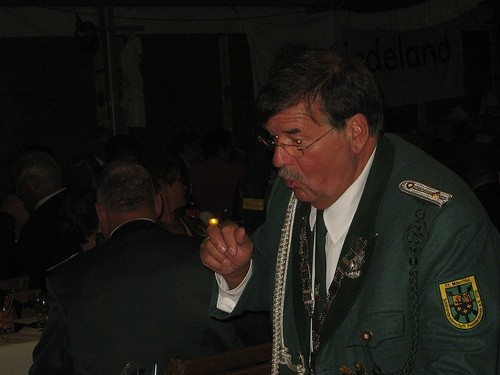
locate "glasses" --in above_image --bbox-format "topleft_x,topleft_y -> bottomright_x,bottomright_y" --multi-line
257,118 -> 346,158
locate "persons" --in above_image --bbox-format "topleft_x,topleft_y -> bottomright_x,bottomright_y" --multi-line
30,157 -> 273,375
0,127 -> 277,335
386,74 -> 500,230
199,44 -> 500,375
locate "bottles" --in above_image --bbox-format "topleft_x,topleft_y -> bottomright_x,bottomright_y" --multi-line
186,182 -> 199,217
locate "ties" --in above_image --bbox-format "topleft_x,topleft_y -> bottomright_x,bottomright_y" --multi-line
314,208 -> 329,351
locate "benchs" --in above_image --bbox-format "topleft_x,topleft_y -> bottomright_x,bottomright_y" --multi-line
160,342 -> 272,375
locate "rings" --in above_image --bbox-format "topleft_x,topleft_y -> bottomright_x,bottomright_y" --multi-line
204,236 -> 210,244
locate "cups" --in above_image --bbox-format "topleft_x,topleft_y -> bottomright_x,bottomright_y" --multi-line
119,356 -> 188,375
